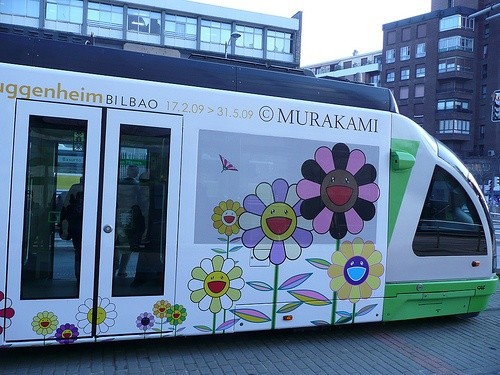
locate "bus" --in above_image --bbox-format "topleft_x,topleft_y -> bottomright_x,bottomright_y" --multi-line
0,22 -> 499,350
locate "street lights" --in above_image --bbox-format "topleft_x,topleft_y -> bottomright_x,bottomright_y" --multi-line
224,32 -> 241,58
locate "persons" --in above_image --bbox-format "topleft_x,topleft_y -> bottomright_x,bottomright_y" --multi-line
114,162 -> 150,280
118,204 -> 146,244
60,175 -> 84,285
